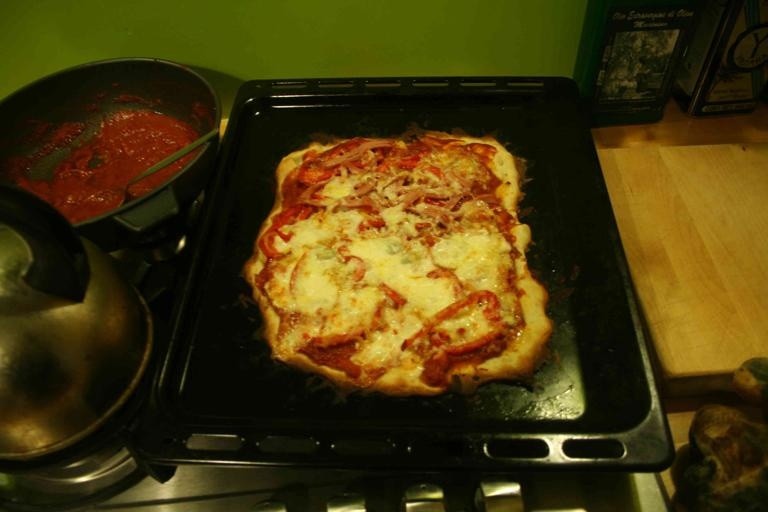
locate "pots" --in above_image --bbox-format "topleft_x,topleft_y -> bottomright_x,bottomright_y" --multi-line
135,72 -> 678,512
0,57 -> 223,254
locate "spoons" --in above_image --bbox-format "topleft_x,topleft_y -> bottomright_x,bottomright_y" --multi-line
95,122 -> 221,213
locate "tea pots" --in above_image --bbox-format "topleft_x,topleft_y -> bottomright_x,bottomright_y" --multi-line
0,179 -> 157,463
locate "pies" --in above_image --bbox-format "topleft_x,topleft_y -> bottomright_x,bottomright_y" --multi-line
239,133 -> 555,400
